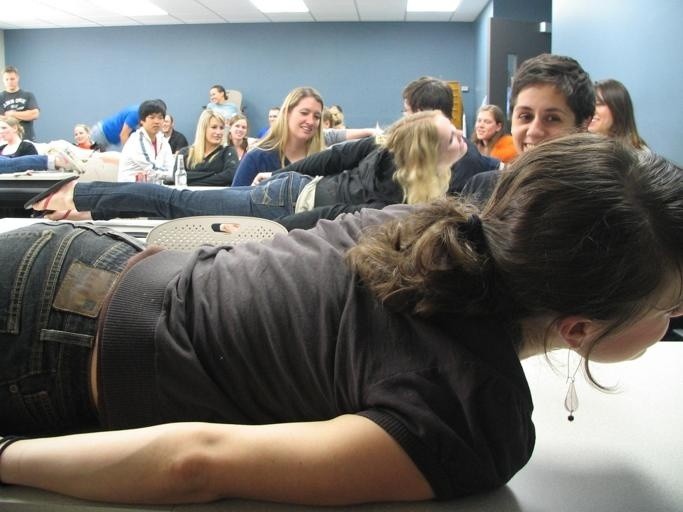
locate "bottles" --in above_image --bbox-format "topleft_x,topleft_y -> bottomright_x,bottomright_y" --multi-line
175,155 -> 187,191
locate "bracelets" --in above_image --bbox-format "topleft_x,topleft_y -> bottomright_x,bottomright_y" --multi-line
0,435 -> 29,457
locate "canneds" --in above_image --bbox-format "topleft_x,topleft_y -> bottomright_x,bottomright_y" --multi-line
135,173 -> 147,183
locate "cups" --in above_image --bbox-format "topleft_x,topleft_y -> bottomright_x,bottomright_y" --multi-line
135,172 -> 163,186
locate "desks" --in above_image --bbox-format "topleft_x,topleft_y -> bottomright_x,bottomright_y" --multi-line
0,171 -> 80,202
0,341 -> 683,512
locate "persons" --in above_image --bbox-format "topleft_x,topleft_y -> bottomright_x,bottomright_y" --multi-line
173,84 -> 345,188
0,136 -> 683,512
0,66 -> 188,184
24,110 -> 465,222
402,54 -> 646,193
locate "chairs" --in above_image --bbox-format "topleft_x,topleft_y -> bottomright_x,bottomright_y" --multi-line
145,215 -> 287,249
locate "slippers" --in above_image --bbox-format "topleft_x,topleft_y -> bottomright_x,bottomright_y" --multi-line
31,210 -> 71,221
23,176 -> 80,211
